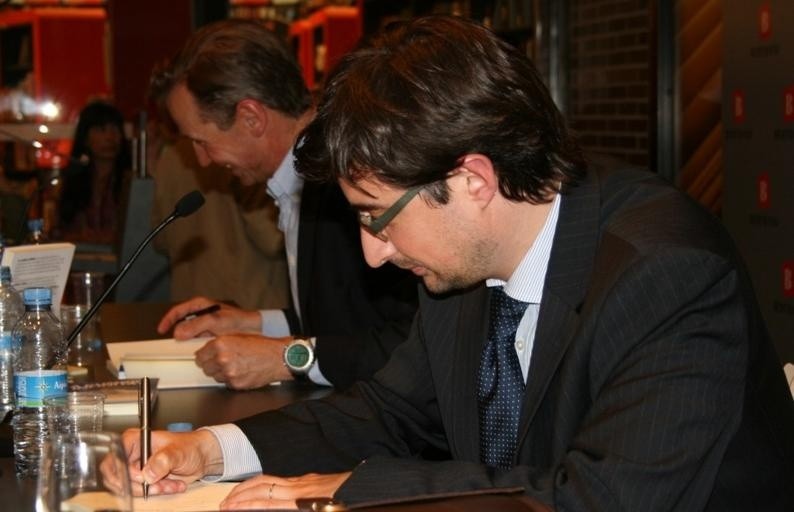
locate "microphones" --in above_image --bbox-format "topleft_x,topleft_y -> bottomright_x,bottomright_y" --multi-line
44,188 -> 207,367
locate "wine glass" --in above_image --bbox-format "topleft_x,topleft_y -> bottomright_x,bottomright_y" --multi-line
57,304 -> 95,370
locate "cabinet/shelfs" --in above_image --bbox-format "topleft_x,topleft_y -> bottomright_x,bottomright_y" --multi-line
288,4 -> 365,95
1,6 -> 115,174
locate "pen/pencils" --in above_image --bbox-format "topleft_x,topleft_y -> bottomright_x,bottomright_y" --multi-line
140,379 -> 153,498
179,304 -> 222,320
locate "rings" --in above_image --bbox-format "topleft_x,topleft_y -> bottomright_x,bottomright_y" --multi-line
266,484 -> 278,498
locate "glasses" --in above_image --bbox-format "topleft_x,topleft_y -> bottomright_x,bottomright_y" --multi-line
360,185 -> 425,242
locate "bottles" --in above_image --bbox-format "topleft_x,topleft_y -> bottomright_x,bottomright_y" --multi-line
0,265 -> 26,404
9,286 -> 71,477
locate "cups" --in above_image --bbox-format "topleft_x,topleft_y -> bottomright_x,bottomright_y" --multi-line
34,431 -> 134,512
44,392 -> 105,461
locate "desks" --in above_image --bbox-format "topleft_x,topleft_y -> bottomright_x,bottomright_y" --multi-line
2,300 -> 527,511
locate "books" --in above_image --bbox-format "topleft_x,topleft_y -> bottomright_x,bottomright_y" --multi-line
104,334 -> 282,388
1,242 -> 76,325
63,379 -> 159,419
60,480 -> 328,512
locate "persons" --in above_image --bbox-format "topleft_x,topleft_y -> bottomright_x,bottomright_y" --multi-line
146,62 -> 289,311
98,16 -> 792,512
2,102 -> 131,304
147,17 -> 451,393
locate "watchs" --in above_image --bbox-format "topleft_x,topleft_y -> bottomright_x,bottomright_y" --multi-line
283,337 -> 315,382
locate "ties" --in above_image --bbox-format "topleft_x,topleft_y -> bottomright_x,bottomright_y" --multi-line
477,287 -> 529,471
